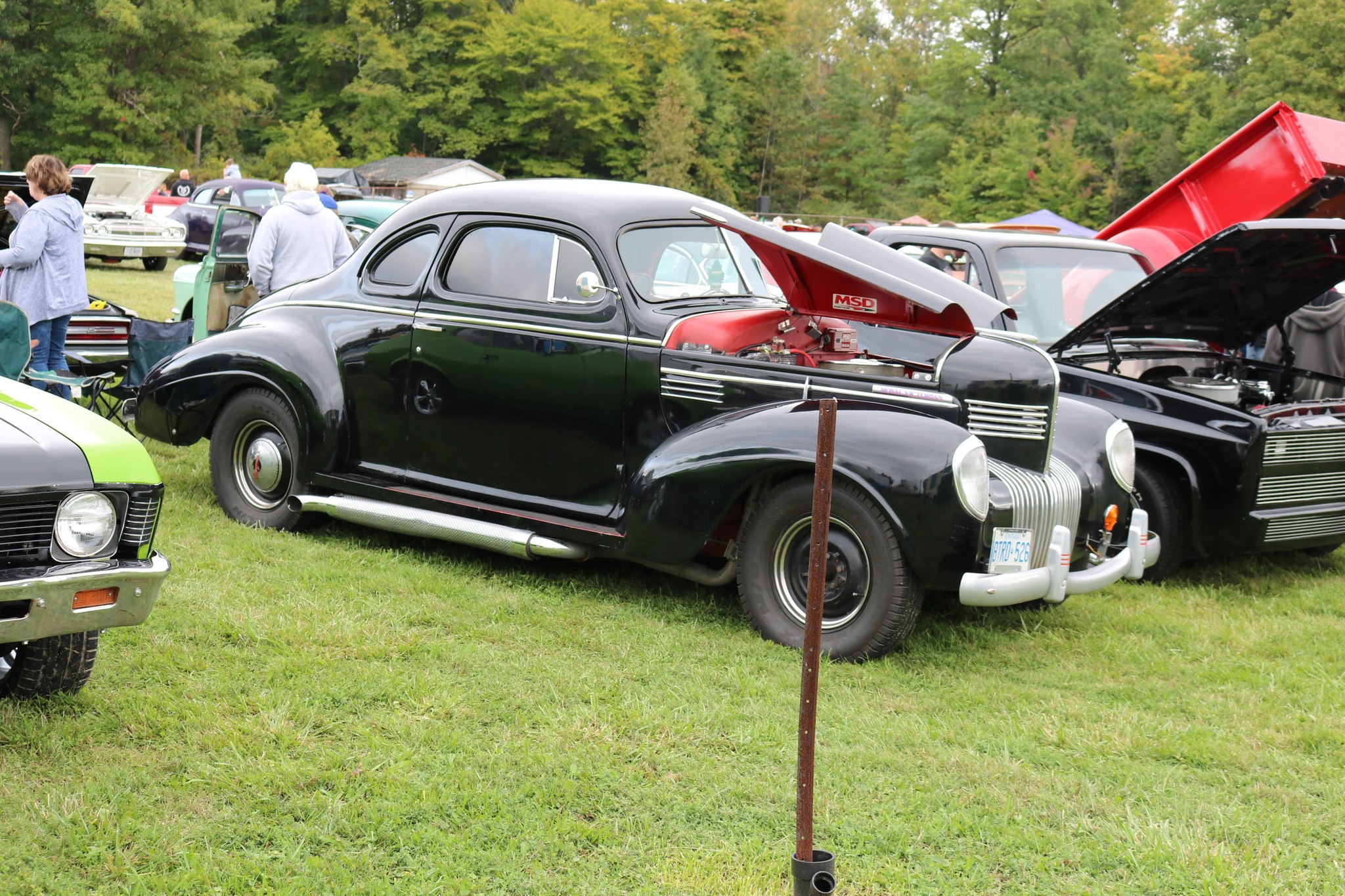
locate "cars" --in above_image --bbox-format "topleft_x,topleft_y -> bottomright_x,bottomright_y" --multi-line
0,162 -> 410,386
0,371 -> 171,706
652,217 -> 1345,585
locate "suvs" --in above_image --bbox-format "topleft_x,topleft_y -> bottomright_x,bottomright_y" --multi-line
142,179 -> 1160,664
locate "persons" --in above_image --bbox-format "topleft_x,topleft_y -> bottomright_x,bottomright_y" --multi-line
0,154 -> 91,403
1262,290 -> 1345,402
223,158 -> 241,179
247,162 -> 360,299
918,221 -> 960,279
159,169 -> 197,198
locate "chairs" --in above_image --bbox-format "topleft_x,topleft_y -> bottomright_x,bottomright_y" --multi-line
0,300 -> 117,421
61,318 -> 195,441
